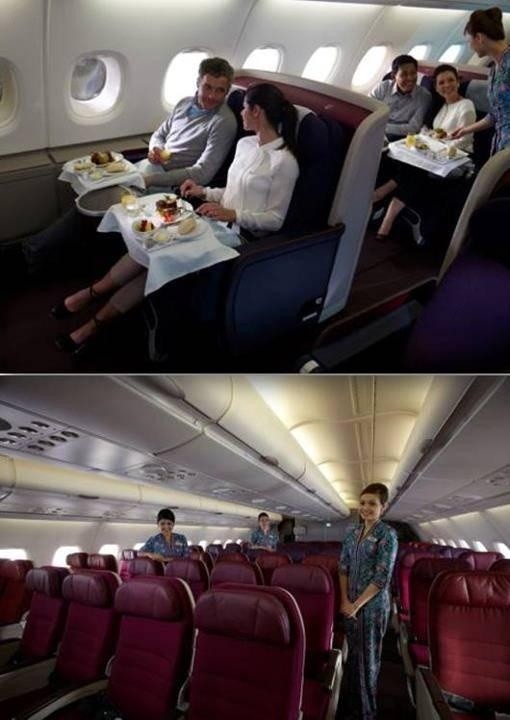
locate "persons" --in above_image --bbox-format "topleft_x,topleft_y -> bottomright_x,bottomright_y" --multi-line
248,513 -> 279,552
373,55 -> 433,181
337,484 -> 398,720
373,65 -> 477,243
50,84 -> 301,354
137,509 -> 190,562
444,7 -> 510,156
62,58 -> 238,305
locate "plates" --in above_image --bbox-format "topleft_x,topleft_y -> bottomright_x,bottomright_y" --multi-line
86,151 -> 124,168
144,199 -> 194,224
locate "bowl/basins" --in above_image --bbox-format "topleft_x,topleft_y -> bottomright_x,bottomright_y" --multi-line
131,217 -> 158,236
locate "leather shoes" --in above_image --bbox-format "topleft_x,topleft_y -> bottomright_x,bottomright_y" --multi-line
51,299 -> 82,351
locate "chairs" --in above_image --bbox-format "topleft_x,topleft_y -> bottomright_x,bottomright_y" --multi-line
397,539 -> 509,718
381,60 -> 510,246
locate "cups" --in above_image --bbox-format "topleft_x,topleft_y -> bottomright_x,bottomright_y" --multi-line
120,190 -> 137,213
406,131 -> 416,148
